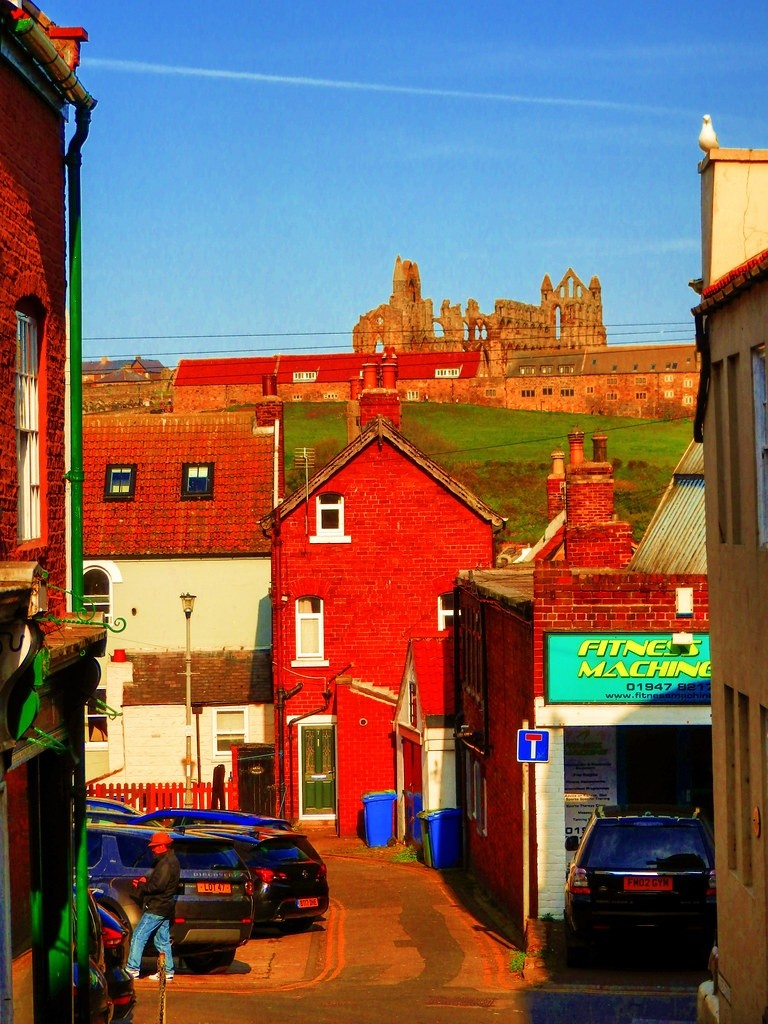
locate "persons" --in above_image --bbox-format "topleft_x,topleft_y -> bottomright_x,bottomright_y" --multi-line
121,832 -> 180,980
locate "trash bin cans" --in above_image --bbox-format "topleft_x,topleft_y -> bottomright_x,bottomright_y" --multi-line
407,791 -> 422,841
416,809 -> 461,869
359,788 -> 397,847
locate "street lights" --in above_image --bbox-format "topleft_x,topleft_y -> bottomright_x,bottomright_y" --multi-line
180,592 -> 198,809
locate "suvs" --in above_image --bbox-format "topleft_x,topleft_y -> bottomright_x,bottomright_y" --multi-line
86,823 -> 254,973
563,806 -> 716,972
176,823 -> 329,938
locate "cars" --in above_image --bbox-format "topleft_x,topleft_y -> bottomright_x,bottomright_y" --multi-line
88,888 -> 138,1024
82,797 -> 294,833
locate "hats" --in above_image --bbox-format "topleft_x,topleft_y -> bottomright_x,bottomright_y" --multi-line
148,832 -> 173,847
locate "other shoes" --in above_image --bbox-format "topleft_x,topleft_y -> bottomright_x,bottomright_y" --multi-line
122,968 -> 140,980
149,972 -> 173,980
